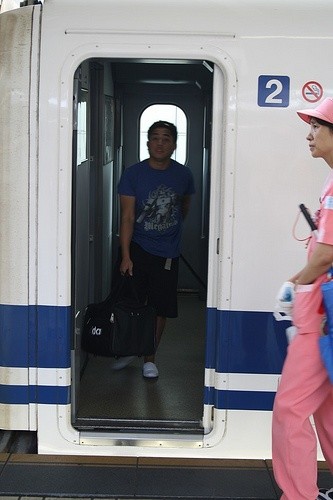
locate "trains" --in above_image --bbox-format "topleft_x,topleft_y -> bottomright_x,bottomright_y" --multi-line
1,0 -> 333,462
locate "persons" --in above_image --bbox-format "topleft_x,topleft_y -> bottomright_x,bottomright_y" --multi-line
117,120 -> 196,381
272,98 -> 333,500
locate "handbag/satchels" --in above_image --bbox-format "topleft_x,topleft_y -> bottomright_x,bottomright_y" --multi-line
80,271 -> 158,359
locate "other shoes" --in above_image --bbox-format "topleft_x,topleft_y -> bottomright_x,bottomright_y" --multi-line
111,356 -> 131,369
142,362 -> 159,378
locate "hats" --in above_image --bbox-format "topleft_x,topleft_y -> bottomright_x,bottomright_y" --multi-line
296,97 -> 333,124
